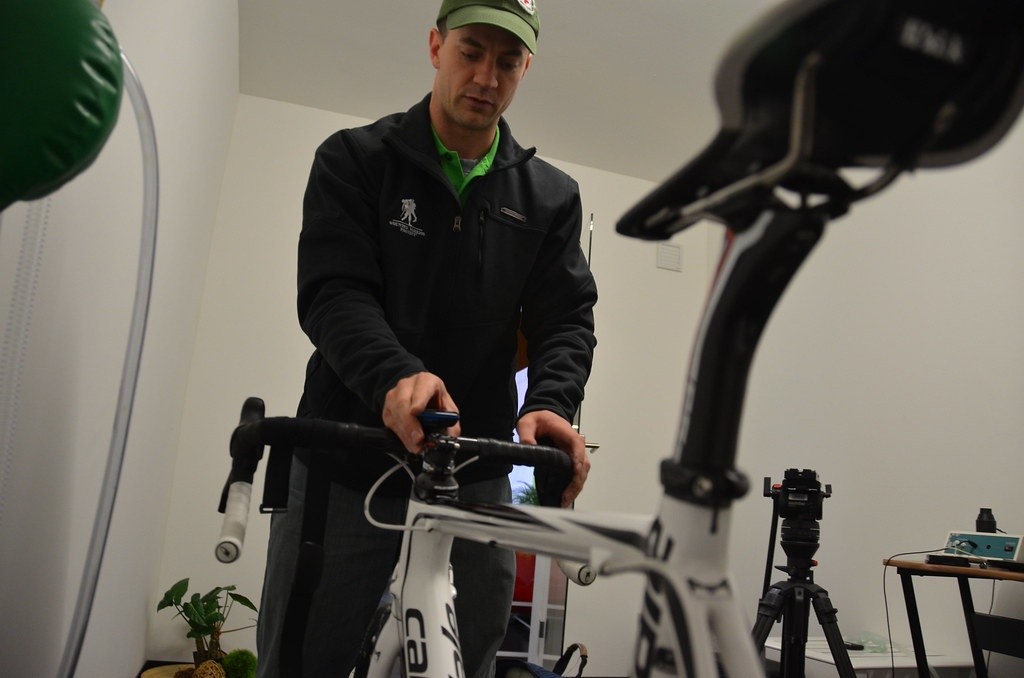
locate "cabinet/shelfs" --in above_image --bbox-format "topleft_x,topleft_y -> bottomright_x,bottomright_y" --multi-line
496,549 -> 552,669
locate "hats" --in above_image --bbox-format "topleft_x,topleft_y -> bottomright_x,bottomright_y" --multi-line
605,0 -> 1023,244
437,0 -> 543,55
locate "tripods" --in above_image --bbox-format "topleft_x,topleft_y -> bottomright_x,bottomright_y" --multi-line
751,468 -> 858,678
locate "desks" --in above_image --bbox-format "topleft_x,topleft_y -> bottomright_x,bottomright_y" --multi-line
882,558 -> 1024,678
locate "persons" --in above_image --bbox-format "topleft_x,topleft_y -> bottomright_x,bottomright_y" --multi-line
256,0 -> 598,678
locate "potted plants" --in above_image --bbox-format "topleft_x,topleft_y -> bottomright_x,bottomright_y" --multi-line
138,577 -> 260,678
